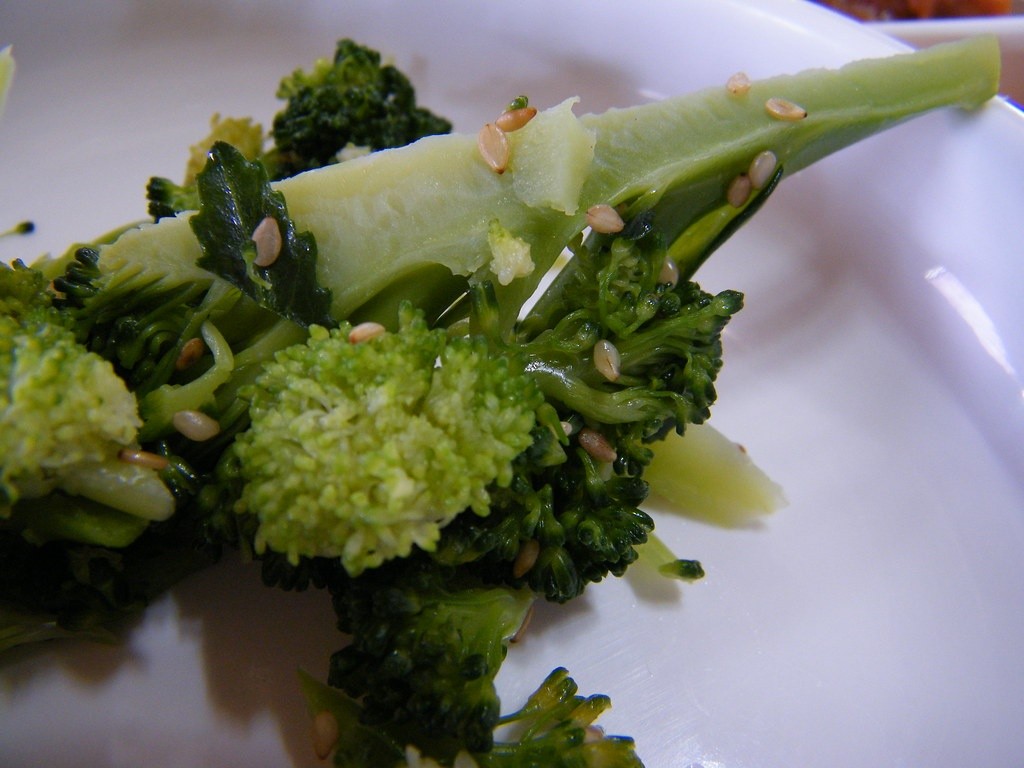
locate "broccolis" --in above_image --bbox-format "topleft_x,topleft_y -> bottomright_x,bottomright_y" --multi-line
0,32 -> 1001,768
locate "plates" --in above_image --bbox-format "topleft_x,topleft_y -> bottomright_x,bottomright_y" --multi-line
0,0 -> 1024,768
